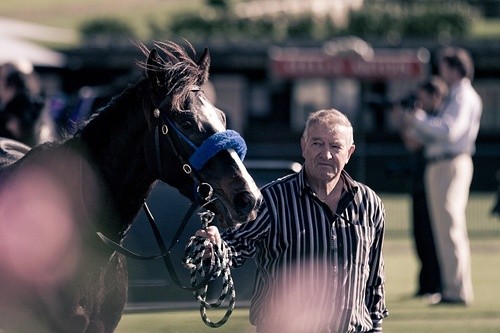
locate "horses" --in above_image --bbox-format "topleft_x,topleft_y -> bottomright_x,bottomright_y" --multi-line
1,35 -> 262,332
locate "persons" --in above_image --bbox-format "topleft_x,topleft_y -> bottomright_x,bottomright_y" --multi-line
395,47 -> 482,305
0,55 -> 448,298
195,108 -> 389,333
